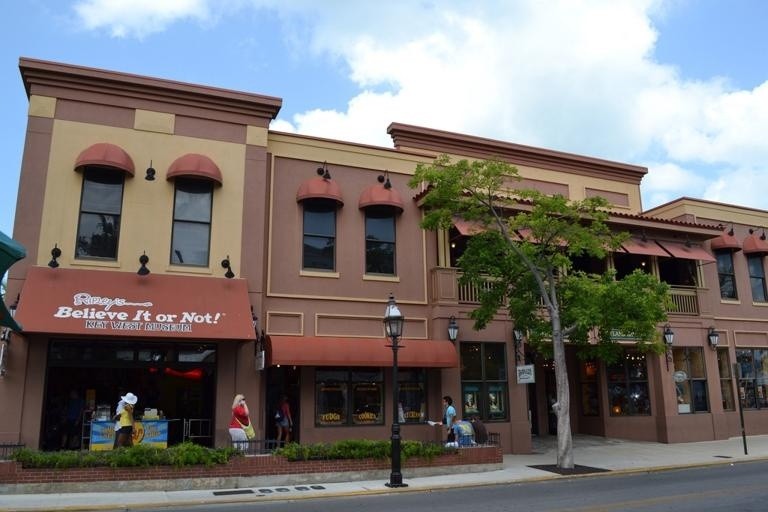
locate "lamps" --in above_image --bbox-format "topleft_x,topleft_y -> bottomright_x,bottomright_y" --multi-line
317,159 -> 331,179
513,324 -> 524,343
145,159 -> 155,181
447,316 -> 460,343
136,250 -> 150,276
718,221 -> 766,240
707,325 -> 719,348
221,254 -> 235,278
47,242 -> 62,267
378,169 -> 392,188
663,322 -> 674,344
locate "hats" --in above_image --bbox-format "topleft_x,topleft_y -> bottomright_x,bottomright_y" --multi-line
121,393 -> 138,405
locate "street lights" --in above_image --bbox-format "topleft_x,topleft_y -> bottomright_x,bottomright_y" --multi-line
382,292 -> 409,489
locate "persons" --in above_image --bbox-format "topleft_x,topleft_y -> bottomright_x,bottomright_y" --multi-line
229,393 -> 255,452
112,392 -> 137,449
275,393 -> 292,450
428,396 -> 488,447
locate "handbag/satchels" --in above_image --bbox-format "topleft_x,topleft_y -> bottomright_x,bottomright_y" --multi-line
443,413 -> 447,423
243,422 -> 256,440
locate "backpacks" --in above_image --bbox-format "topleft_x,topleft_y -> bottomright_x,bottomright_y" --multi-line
273,401 -> 286,422
